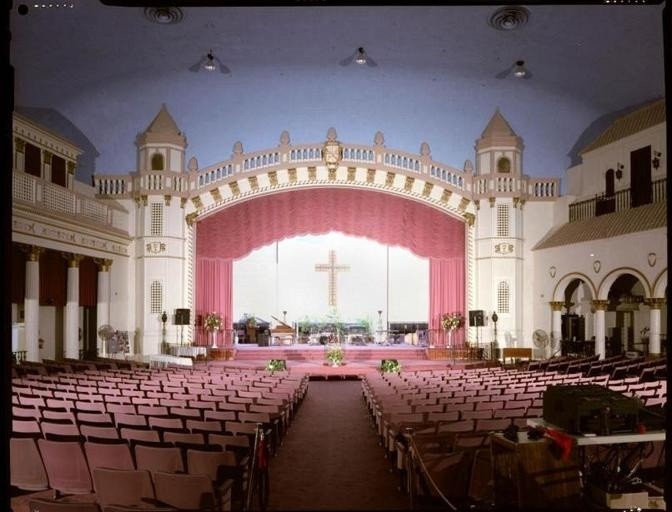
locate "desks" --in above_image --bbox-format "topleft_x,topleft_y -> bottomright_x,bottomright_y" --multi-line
490,431 -> 563,512
560,340 -> 595,357
526,417 -> 666,496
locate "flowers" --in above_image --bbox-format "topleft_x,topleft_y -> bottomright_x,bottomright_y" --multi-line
442,313 -> 464,331
204,311 -> 223,331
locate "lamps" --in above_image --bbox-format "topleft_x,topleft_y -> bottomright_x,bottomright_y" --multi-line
189,50 -> 230,75
510,60 -> 533,79
339,48 -> 378,68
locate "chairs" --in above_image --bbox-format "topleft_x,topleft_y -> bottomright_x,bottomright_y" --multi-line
526,354 -> 667,417
409,432 -> 491,504
362,362 -> 526,431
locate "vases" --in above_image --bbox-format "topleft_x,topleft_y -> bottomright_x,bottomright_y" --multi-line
447,331 -> 452,348
212,332 -> 218,349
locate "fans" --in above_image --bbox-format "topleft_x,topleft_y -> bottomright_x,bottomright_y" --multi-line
533,330 -> 549,360
98,325 -> 114,358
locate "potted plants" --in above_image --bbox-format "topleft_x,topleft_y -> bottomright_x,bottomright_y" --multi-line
326,347 -> 342,367
